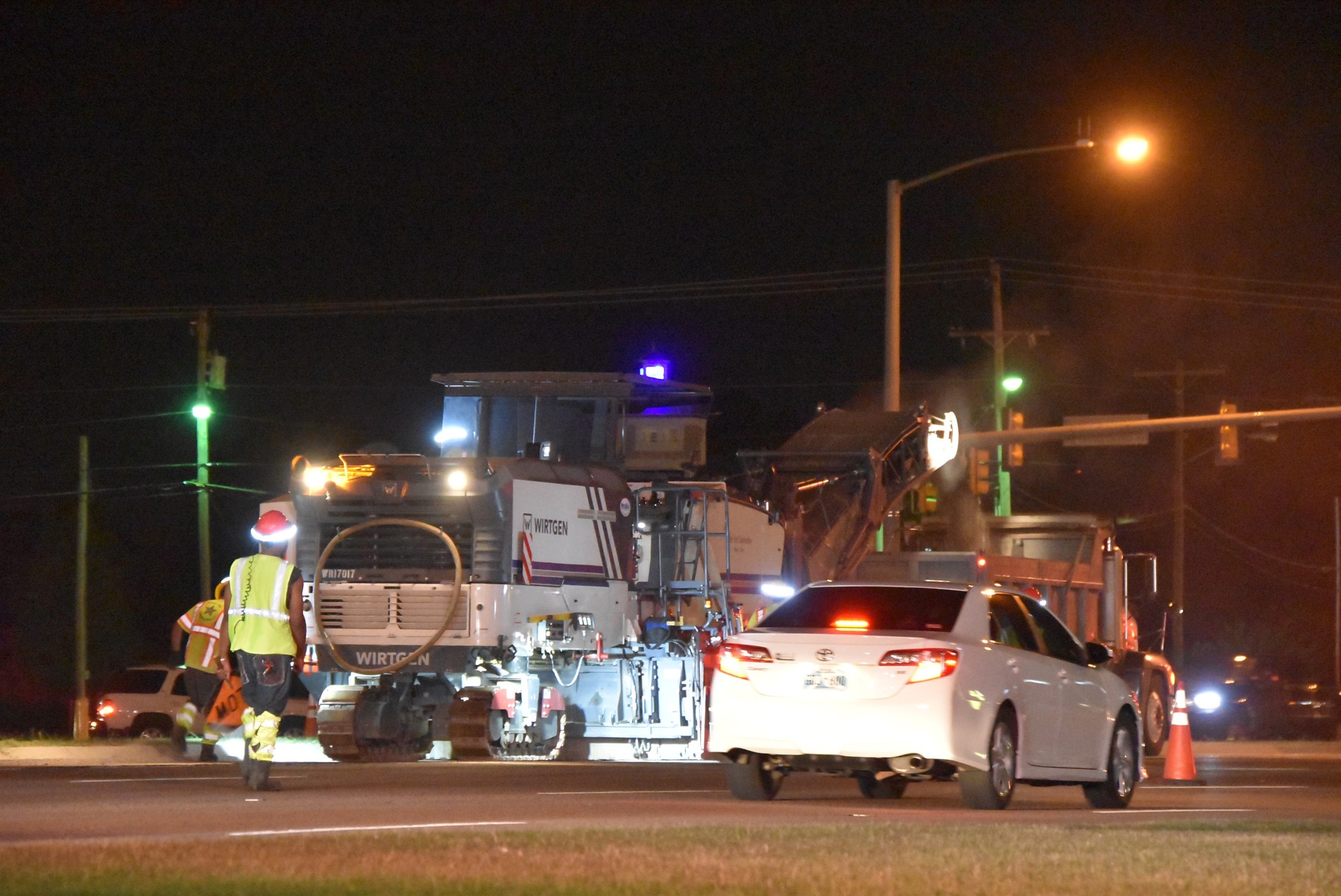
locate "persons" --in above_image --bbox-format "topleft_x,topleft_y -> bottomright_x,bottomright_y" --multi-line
214,510 -> 306,790
172,577 -> 230,763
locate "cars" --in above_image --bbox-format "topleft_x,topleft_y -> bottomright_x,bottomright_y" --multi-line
707,577 -> 1146,811
1182,656 -> 1341,743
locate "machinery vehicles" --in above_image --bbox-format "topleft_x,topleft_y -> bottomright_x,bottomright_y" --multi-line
257,358 -> 959,762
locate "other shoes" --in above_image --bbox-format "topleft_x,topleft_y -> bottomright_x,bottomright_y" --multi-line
169,729 -> 188,753
241,757 -> 252,777
249,777 -> 281,791
199,752 -> 218,761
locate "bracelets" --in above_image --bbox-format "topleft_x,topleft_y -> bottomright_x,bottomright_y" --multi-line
220,658 -> 228,661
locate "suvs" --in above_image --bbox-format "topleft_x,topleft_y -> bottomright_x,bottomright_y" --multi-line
95,661 -> 309,740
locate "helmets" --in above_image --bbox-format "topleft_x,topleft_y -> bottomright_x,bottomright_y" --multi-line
215,577 -> 230,599
251,510 -> 297,543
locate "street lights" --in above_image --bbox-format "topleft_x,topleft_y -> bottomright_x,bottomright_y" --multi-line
303,691 -> 318,736
1162,680 -> 1197,781
881,131 -> 1150,558
